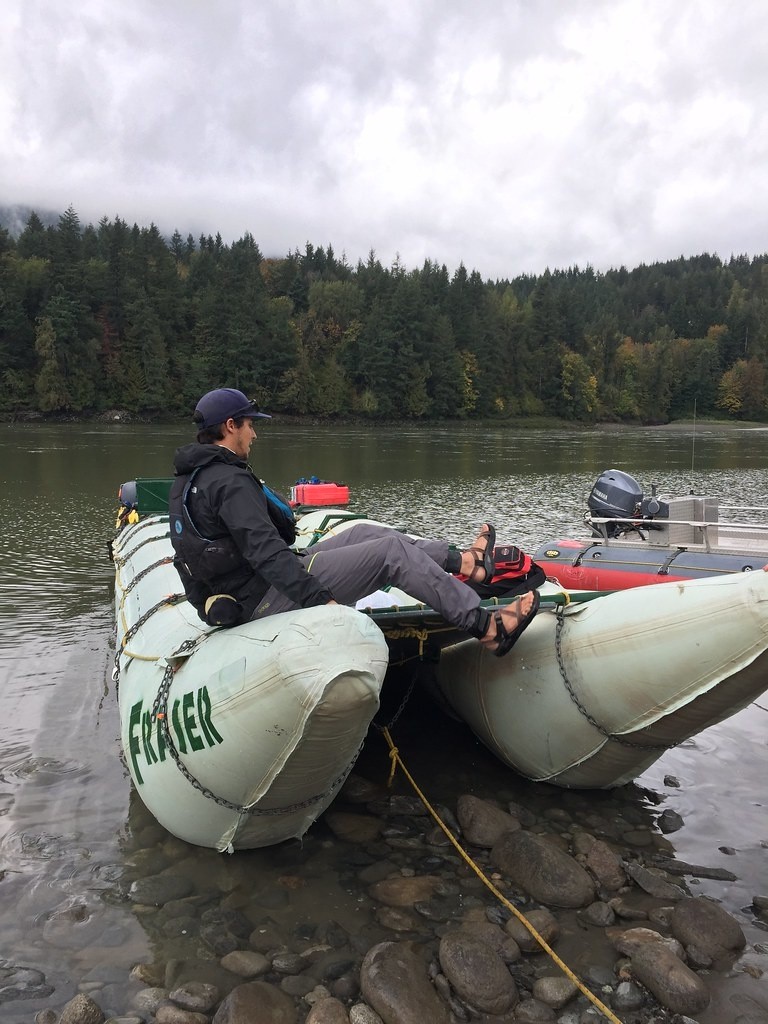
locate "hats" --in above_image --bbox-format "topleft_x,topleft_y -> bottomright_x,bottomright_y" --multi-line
194,388 -> 272,430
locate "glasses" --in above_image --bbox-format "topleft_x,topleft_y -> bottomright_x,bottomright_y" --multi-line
227,398 -> 260,419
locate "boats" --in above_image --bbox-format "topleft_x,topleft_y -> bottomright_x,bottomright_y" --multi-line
529,470 -> 768,591
106,476 -> 768,856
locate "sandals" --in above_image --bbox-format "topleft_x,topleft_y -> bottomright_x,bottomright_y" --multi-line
479,589 -> 540,658
463,524 -> 496,585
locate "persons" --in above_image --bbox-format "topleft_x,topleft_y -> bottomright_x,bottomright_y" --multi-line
166,388 -> 540,657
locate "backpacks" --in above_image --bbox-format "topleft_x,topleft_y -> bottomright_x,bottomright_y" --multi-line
450,544 -> 546,597
